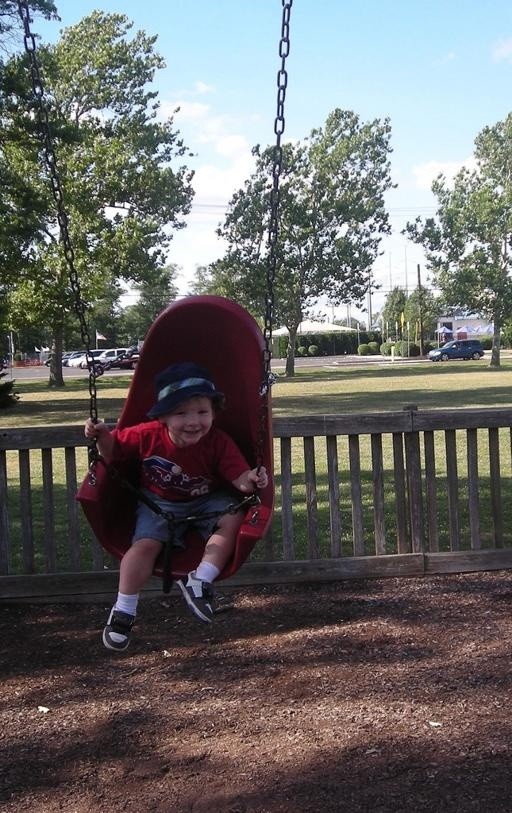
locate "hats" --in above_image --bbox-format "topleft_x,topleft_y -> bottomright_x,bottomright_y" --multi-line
146,361 -> 225,420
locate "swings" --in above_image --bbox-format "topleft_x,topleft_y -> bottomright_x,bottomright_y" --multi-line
17,0 -> 291,580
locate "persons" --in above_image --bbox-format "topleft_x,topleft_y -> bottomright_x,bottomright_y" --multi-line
84,361 -> 269,653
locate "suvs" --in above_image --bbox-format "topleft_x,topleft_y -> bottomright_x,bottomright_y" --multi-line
43,348 -> 140,369
427,339 -> 484,362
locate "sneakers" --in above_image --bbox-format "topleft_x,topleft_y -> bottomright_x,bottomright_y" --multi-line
176,570 -> 215,625
102,602 -> 137,651
